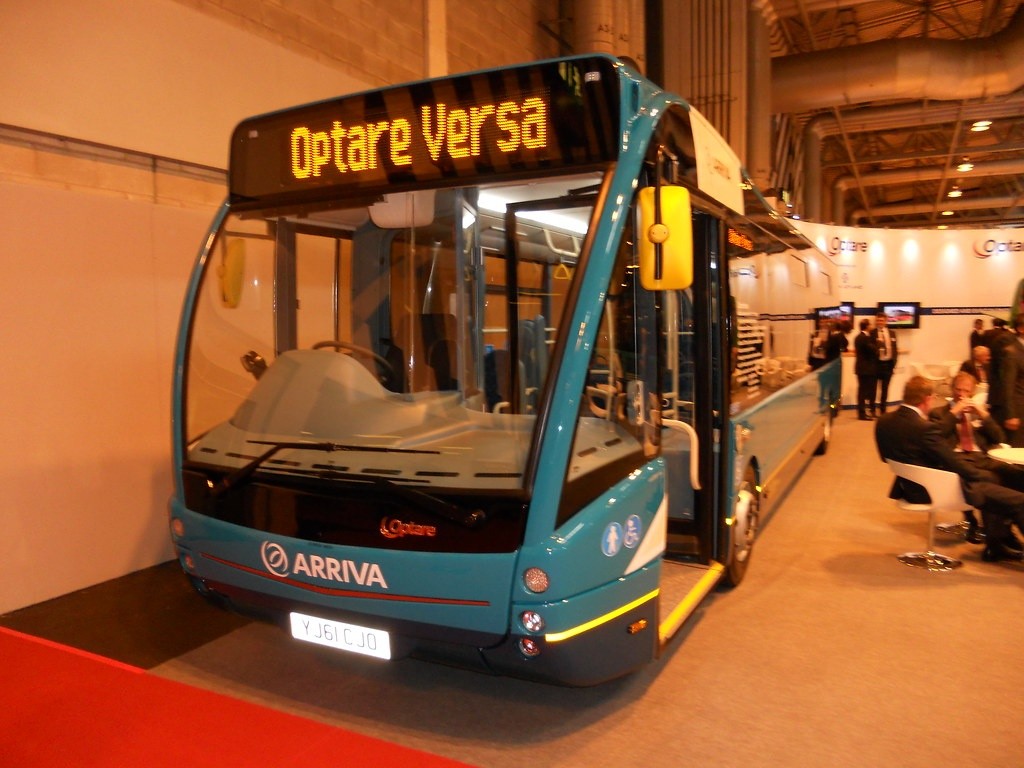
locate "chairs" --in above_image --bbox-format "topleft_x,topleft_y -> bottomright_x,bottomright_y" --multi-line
884,456 -> 977,572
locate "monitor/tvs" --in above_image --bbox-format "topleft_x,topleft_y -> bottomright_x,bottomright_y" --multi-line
877,302 -> 919,328
815,308 -> 854,330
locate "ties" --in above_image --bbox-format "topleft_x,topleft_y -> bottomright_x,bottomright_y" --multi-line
961,413 -> 973,452
880,330 -> 887,356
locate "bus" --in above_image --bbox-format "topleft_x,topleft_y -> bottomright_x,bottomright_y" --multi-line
167,52 -> 842,687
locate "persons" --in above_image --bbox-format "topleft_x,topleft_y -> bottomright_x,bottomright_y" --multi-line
874,375 -> 1024,562
929,371 -> 1007,544
841,312 -> 898,421
958,317 -> 1024,448
808,315 -> 841,415
731,345 -> 747,405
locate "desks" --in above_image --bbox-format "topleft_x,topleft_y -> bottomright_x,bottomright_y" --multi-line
987,447 -> 1024,492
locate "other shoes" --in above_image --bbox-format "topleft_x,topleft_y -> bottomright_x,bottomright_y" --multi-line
981,546 -> 1022,560
860,414 -> 873,421
